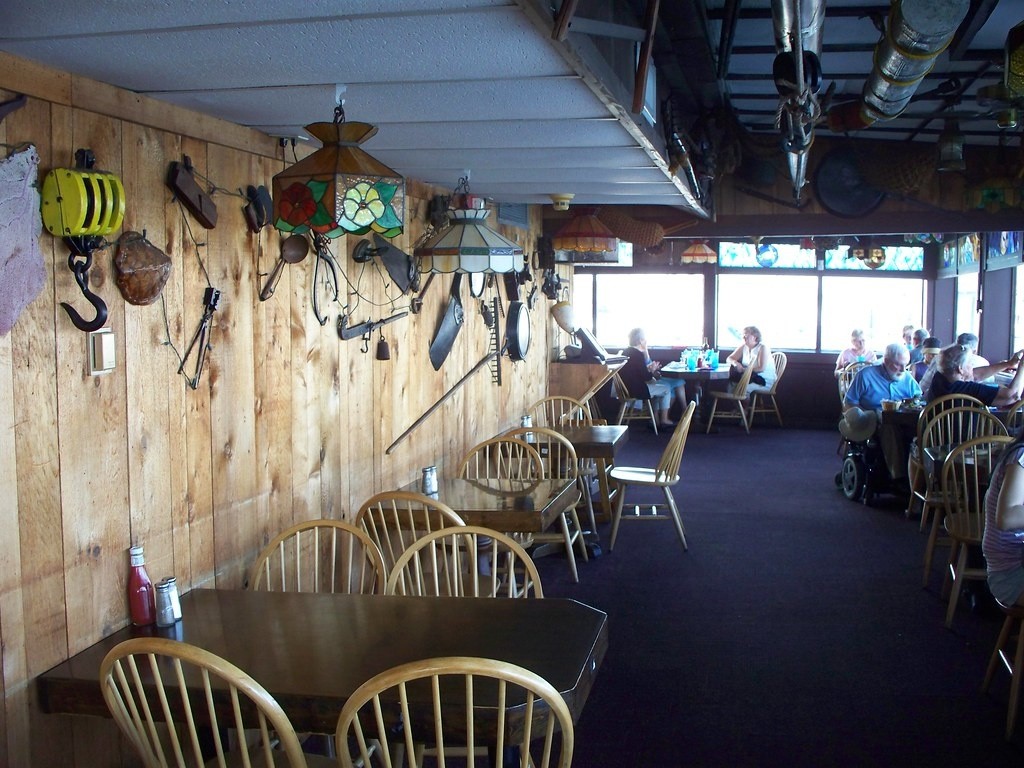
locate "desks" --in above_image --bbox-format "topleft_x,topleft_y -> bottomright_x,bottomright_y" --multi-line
549,358 -> 628,480
31,586 -> 609,768
876,405 -> 1024,425
660,362 -> 734,436
923,443 -> 990,555
363,477 -> 580,535
484,426 -> 630,525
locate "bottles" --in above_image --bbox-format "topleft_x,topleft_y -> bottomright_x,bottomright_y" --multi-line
521,416 -> 528,435
703,337 -> 709,353
527,415 -> 532,428
698,358 -> 702,367
162,577 -> 182,621
128,547 -> 156,627
422,468 -> 432,495
429,466 -> 437,493
155,582 -> 175,628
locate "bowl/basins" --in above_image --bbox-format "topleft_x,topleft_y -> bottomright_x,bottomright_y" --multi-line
881,402 -> 901,411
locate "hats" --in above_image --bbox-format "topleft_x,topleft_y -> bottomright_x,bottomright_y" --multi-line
839,407 -> 876,442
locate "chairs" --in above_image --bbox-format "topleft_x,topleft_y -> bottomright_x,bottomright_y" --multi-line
334,655 -> 576,768
610,400 -> 696,552
103,639 -> 329,768
246,394 -> 608,599
706,355 -> 757,436
741,351 -> 787,431
611,373 -> 659,436
835,361 -> 1024,746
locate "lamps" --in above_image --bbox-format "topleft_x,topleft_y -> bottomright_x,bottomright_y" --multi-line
412,175 -> 524,273
680,238 -> 716,264
548,214 -> 617,251
271,100 -> 405,239
848,235 -> 886,260
925,103 -> 981,172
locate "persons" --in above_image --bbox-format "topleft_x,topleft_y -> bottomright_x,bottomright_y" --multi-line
908,344 -> 1024,517
617,328 -> 678,428
842,343 -> 922,494
981,425 -> 1024,607
726,326 -> 777,426
642,340 -> 687,424
919,332 -> 995,396
834,329 -> 877,379
911,338 -> 941,384
903,325 -> 916,349
907,329 -> 931,371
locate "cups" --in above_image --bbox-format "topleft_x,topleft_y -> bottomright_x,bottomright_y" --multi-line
712,353 -> 718,369
687,355 -> 695,370
903,398 -> 912,405
857,356 -> 865,362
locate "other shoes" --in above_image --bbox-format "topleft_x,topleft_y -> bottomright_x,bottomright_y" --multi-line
661,419 -> 676,429
648,421 -> 660,431
894,479 -> 909,494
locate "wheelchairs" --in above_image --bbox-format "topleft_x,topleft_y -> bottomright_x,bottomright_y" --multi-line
835,409 -> 927,501
